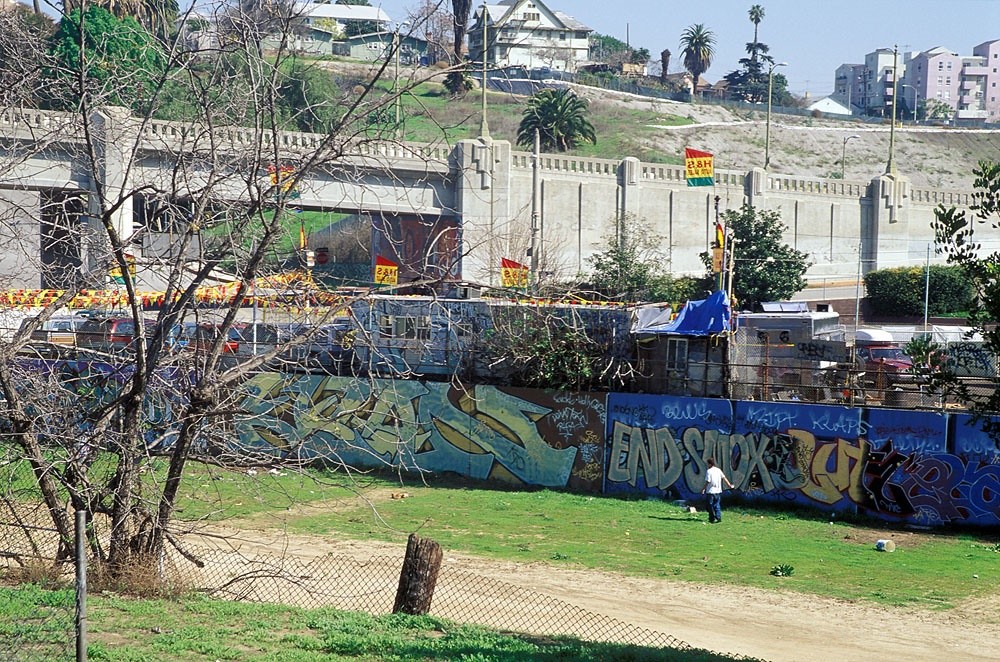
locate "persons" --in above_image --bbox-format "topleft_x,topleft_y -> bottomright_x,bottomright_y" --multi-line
701,458 -> 734,524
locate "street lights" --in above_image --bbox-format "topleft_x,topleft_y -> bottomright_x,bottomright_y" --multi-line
764,62 -> 789,169
842,135 -> 861,195
394,20 -> 410,140
902,84 -> 917,121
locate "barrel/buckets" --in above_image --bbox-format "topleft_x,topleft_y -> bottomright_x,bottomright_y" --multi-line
877,539 -> 895,552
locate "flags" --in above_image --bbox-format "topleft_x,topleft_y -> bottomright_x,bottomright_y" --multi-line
501,257 -> 529,295
684,147 -> 715,191
267,163 -> 301,205
374,254 -> 400,295
715,223 -> 725,293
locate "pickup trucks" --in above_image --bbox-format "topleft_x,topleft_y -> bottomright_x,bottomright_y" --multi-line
854,328 -> 948,390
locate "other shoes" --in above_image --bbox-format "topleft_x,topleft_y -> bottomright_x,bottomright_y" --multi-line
716,519 -> 721,523
